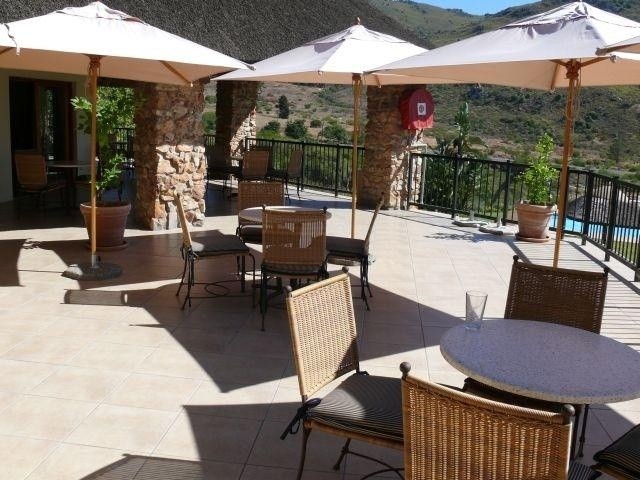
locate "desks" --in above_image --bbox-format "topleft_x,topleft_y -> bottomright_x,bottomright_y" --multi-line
439,318 -> 640,407
47,160 -> 96,211
238,206 -> 332,290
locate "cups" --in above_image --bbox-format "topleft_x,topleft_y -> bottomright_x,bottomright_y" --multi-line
464,290 -> 488,333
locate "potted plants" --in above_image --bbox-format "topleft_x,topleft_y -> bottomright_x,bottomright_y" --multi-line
75,84 -> 143,250
514,131 -> 561,241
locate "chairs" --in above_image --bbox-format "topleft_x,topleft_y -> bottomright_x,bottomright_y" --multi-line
176,193 -> 255,313
285,265 -> 404,478
237,180 -> 287,291
76,134 -> 135,212
260,189 -> 385,331
204,135 -> 302,203
15,151 -> 66,213
507,251 -> 611,458
399,361 -> 577,479
